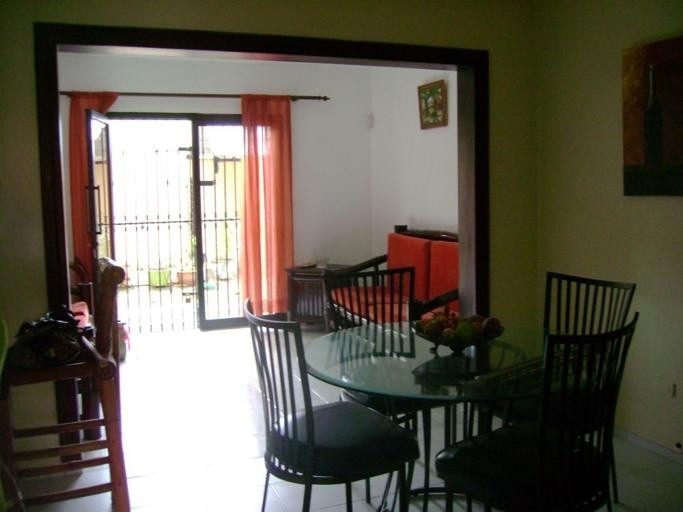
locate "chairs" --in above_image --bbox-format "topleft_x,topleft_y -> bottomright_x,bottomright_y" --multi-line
244,265 -> 418,511
434,264 -> 638,511
0,256 -> 132,512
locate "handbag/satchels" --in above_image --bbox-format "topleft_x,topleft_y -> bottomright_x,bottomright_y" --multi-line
4,301 -> 105,381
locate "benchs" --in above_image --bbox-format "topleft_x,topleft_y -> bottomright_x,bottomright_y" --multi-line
321,222 -> 460,325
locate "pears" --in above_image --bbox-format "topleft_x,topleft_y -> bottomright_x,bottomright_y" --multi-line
456,321 -> 475,338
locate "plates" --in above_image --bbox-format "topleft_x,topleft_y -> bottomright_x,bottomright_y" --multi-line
413,355 -> 490,388
411,321 -> 504,353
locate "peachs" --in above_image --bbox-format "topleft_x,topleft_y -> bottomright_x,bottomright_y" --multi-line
480,317 -> 501,332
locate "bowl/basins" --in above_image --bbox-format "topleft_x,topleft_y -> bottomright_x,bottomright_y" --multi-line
394,225 -> 408,234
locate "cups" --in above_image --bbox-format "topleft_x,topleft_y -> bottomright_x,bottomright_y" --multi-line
315,255 -> 327,269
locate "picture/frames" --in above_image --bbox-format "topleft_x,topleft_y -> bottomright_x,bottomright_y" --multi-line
416,80 -> 449,130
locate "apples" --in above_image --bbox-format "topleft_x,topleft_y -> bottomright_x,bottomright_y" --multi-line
440,327 -> 455,338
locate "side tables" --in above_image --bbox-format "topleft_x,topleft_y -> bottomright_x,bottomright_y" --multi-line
302,323 -> 532,511
